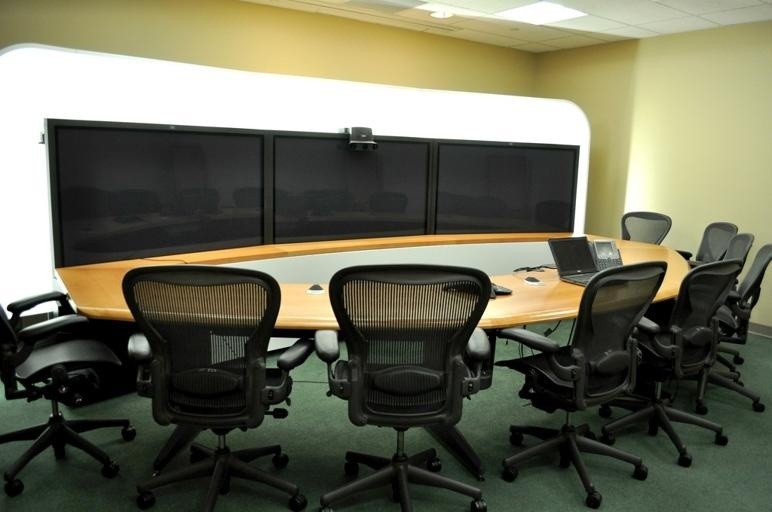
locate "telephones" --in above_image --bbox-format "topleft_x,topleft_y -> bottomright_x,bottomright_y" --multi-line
587,240 -> 623,271
443,277 -> 512,298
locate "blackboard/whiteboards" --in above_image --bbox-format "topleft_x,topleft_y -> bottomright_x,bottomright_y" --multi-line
43,119 -> 590,270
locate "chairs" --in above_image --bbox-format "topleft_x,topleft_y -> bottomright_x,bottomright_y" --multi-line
675,222 -> 738,269
121,265 -> 315,512
316,264 -> 494,511
701,242 -> 772,385
493,259 -> 667,510
601,259 -> 743,467
0,288 -> 137,497
720,232 -> 754,309
620,212 -> 671,248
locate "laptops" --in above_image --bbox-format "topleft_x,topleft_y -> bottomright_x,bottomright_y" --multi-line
548,236 -> 625,288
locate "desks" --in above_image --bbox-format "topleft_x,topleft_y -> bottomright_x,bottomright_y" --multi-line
54,231 -> 689,339
